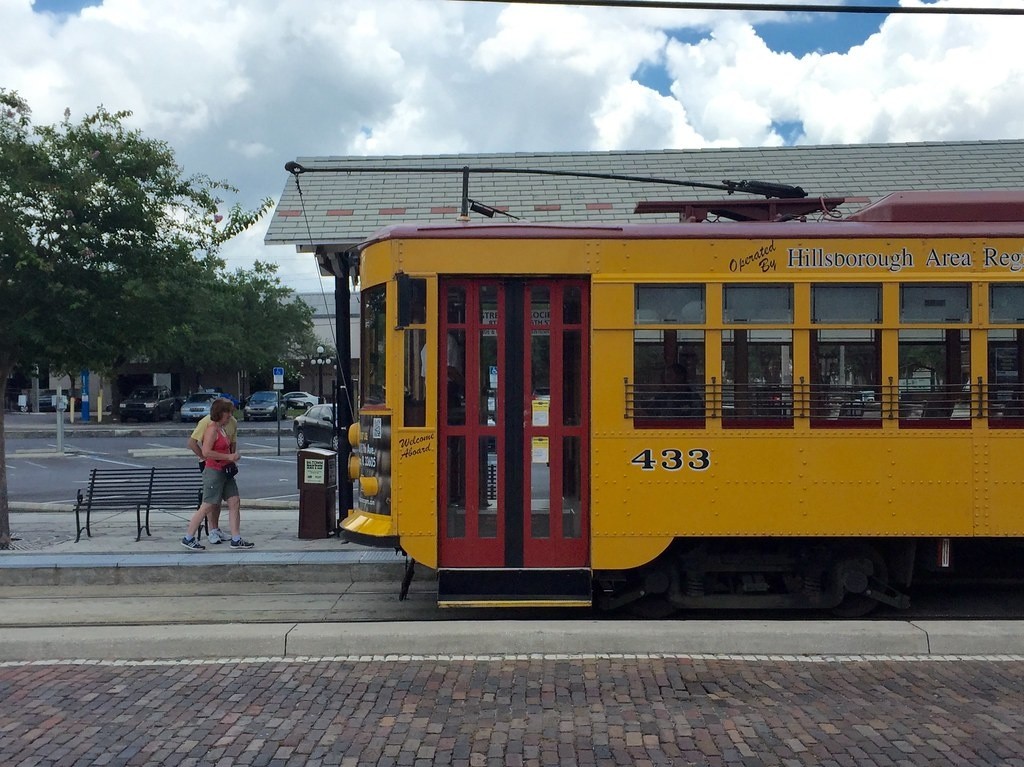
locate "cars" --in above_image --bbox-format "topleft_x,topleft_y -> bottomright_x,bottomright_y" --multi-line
11,389 -> 82,413
179,392 -> 222,422
284,391 -> 326,411
242,391 -> 289,422
293,403 -> 340,451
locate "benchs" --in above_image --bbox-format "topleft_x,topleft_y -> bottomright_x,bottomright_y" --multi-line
73,467 -> 210,544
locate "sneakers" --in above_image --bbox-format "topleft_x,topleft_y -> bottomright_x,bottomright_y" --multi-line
181,536 -> 205,550
207,531 -> 221,545
215,529 -> 231,541
230,538 -> 255,549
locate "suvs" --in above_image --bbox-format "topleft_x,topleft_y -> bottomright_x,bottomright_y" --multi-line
118,385 -> 177,422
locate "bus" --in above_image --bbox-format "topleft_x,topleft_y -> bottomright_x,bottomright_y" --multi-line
335,186 -> 1024,615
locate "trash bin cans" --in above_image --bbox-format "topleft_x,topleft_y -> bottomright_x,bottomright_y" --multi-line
294,447 -> 336,539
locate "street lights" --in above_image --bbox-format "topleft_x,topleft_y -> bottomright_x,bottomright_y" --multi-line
311,346 -> 332,405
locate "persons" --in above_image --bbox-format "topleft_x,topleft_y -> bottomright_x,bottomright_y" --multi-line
181,397 -> 254,550
188,393 -> 238,544
665,364 -> 703,420
647,362 -> 676,413
420,314 -> 466,511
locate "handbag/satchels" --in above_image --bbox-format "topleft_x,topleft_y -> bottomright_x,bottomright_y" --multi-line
224,463 -> 238,477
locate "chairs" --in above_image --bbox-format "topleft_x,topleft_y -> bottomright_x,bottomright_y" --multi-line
820,393 -> 976,423
